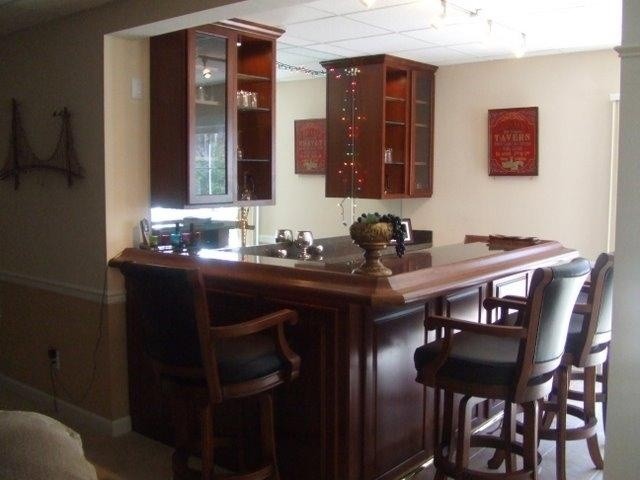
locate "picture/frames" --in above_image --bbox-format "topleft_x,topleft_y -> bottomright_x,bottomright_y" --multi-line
487,106 -> 539,177
294,118 -> 327,175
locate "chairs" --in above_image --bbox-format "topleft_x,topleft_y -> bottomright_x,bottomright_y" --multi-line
120,260 -> 304,480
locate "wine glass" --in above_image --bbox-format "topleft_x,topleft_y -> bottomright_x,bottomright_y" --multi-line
274,229 -> 314,261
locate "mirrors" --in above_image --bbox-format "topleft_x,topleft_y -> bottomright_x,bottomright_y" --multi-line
153,41 -> 403,256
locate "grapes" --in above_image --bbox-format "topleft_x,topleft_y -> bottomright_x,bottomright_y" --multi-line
358,212 -> 408,258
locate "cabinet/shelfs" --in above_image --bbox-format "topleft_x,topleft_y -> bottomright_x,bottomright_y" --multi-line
319,57 -> 355,198
151,17 -> 286,210
354,53 -> 440,201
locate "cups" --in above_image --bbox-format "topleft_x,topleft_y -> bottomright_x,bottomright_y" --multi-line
149,232 -> 191,248
236,90 -> 259,109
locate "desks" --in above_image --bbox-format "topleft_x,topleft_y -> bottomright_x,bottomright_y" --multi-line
108,233 -> 581,480
